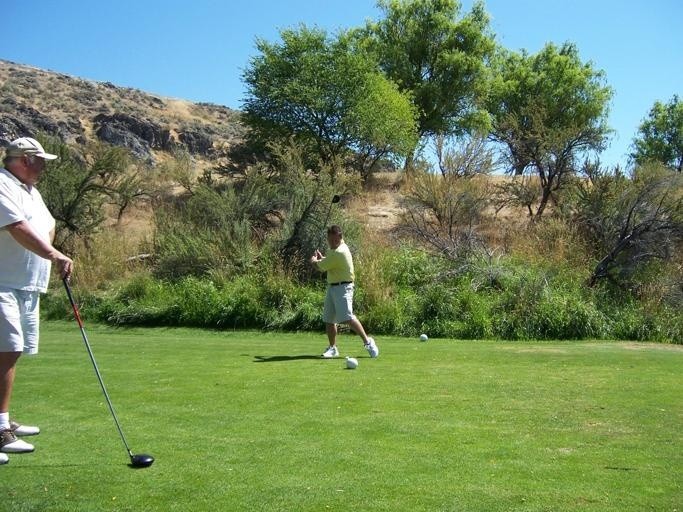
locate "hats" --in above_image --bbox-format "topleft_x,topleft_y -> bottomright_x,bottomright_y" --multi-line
6,137 -> 57,161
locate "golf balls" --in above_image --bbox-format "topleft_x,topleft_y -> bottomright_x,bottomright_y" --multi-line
421,334 -> 428,341
344,356 -> 350,361
346,358 -> 358,369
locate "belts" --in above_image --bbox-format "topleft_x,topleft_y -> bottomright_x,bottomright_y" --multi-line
330,281 -> 352,286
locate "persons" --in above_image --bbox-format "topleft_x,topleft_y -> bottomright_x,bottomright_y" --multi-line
1,136 -> 74,453
309,226 -> 379,359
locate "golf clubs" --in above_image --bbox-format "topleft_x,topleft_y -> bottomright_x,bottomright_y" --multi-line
315,195 -> 340,250
63,277 -> 154,467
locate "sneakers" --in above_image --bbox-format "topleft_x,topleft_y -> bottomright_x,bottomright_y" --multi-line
322,347 -> 339,358
8,420 -> 40,437
364,336 -> 378,358
0,453 -> 9,465
1,427 -> 34,452
